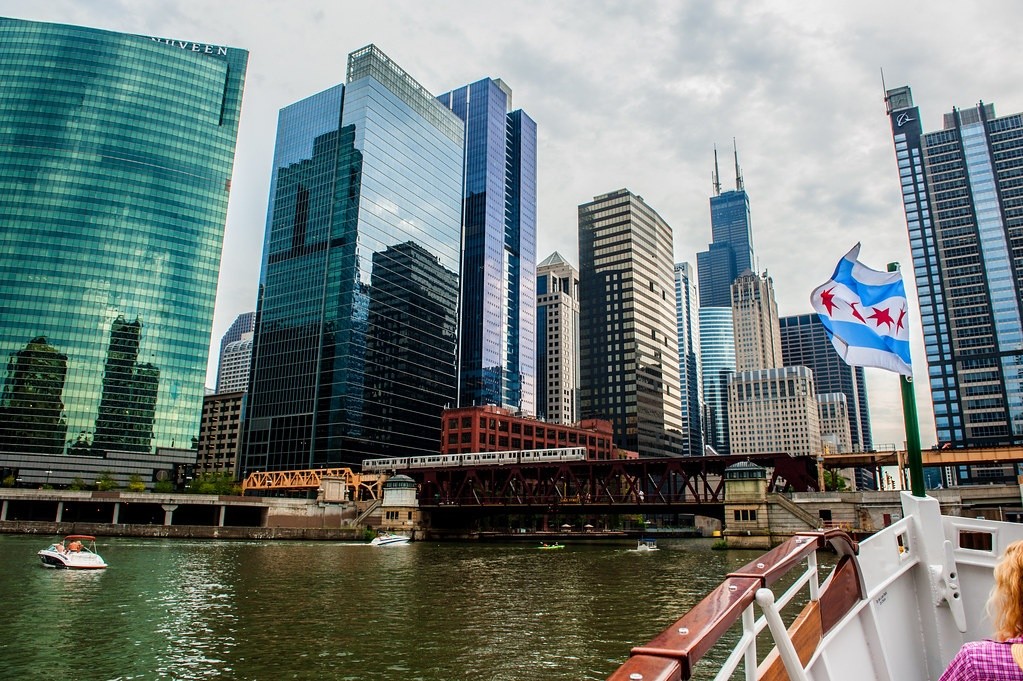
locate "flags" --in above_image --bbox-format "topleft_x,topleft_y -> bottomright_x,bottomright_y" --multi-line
810,242 -> 913,378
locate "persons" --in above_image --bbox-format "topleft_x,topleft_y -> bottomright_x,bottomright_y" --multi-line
938,540 -> 1023,680
51,539 -> 83,553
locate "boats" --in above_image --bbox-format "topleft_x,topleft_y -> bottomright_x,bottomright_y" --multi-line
636,532 -> 661,553
36,533 -> 108,570
370,531 -> 411,547
